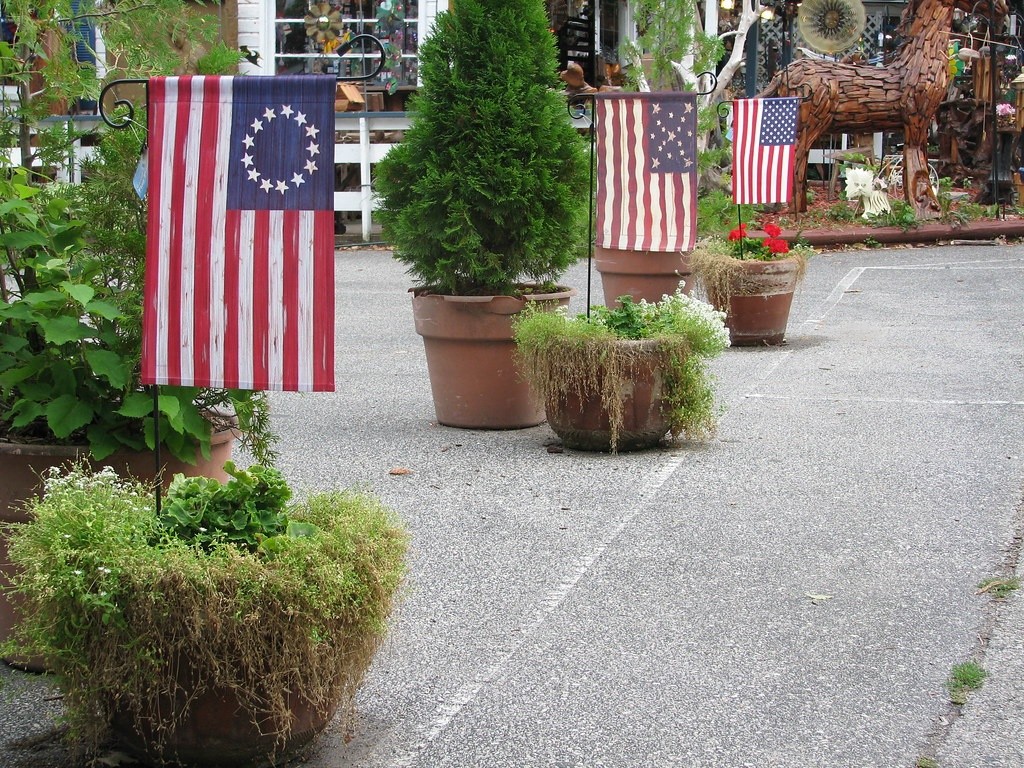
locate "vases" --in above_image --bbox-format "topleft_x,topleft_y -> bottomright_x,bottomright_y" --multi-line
996,114 -> 1018,130
533,336 -> 691,450
697,258 -> 801,345
59,576 -> 384,768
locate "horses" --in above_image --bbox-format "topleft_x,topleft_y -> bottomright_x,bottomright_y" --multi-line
750,0 -> 1008,220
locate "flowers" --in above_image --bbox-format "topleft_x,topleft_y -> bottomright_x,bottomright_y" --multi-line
995,101 -> 1018,117
617,272 -> 734,353
728,216 -> 792,256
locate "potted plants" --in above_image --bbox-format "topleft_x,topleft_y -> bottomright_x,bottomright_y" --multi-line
368,0 -> 601,430
594,0 -> 732,311
0,0 -> 259,675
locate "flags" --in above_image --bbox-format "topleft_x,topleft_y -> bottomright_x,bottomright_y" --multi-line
141,74 -> 339,392
596,91 -> 697,252
733,97 -> 798,205
